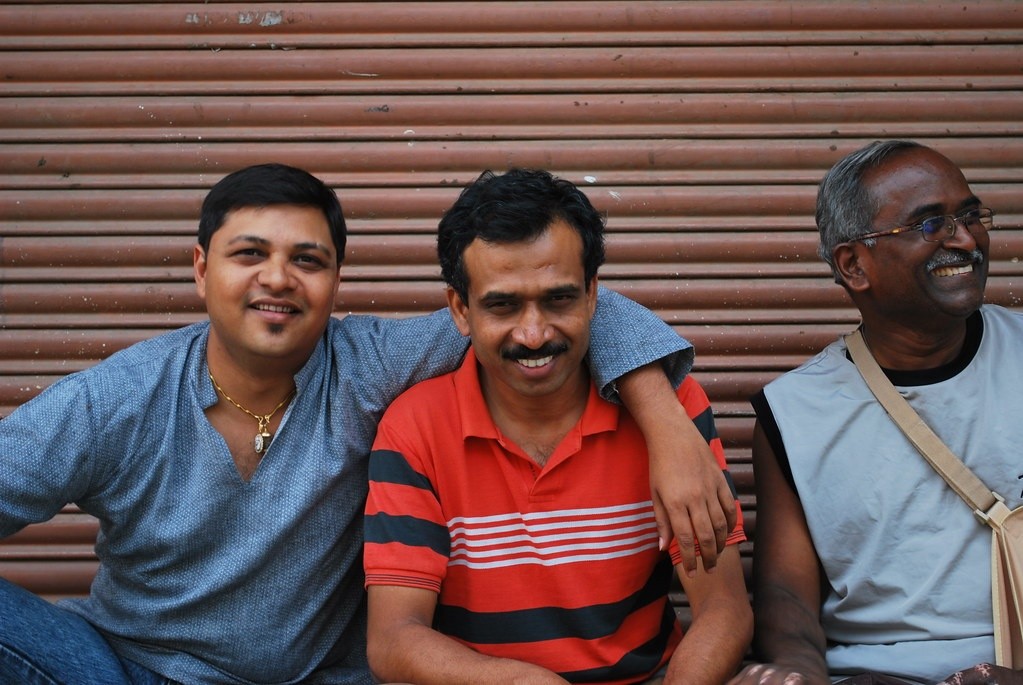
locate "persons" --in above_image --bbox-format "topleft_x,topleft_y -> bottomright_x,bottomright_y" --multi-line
719,139 -> 1022,685
0,161 -> 738,685
361,164 -> 755,685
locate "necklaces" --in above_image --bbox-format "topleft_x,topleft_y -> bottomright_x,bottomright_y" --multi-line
209,370 -> 297,454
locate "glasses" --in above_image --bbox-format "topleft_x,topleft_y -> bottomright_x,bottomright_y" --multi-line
849,207 -> 996,242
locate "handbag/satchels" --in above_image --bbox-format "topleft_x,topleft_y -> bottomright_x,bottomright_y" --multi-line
991,506 -> 1023,669
